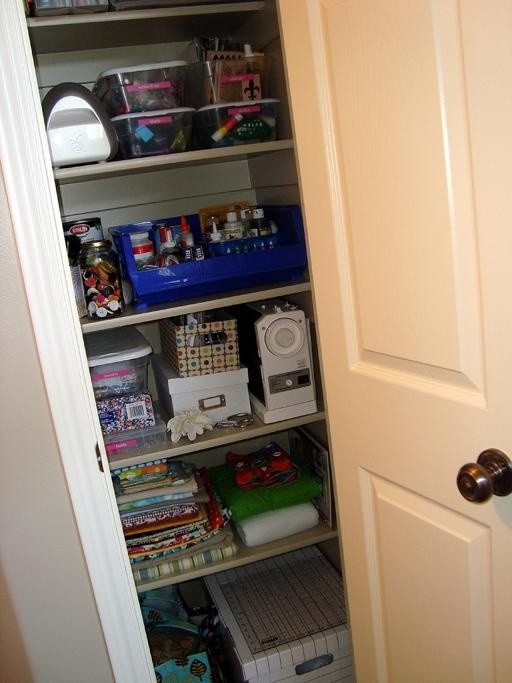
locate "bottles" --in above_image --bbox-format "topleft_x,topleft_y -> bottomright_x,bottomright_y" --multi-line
128,206 -> 278,261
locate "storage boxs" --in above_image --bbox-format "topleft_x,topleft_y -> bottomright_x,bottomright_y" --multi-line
110,108 -> 196,160
96,390 -> 154,438
195,55 -> 273,107
203,546 -> 356,683
193,98 -> 280,148
150,352 -> 250,421
93,60 -> 188,118
159,319 -> 241,378
82,325 -> 155,399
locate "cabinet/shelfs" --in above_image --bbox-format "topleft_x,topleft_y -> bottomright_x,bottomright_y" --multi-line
19,0 -> 357,683
